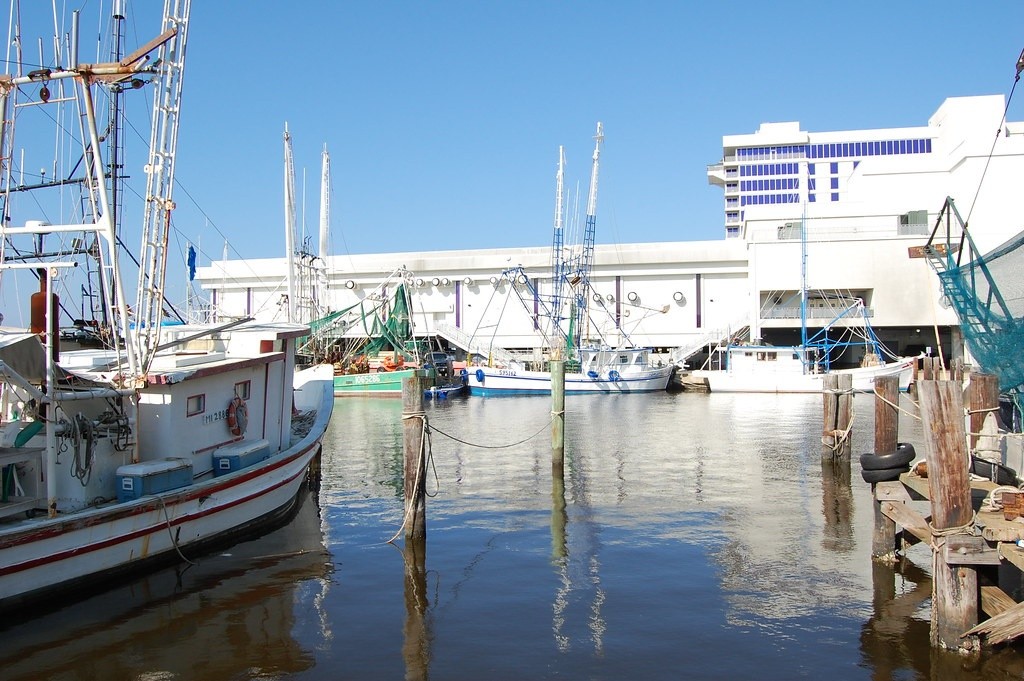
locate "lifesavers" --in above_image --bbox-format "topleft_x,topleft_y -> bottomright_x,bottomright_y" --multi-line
587,370 -> 598,378
227,396 -> 250,436
475,368 -> 485,382
607,370 -> 619,381
460,369 -> 468,383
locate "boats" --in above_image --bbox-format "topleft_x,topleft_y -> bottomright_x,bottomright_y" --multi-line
1,284 -> 108,351
0,0 -> 335,599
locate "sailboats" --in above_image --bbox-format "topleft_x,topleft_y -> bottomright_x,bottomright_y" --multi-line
460,122 -> 673,398
291,143 -> 436,397
677,199 -> 914,392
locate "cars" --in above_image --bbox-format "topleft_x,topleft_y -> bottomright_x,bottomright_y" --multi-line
422,352 -> 455,377
